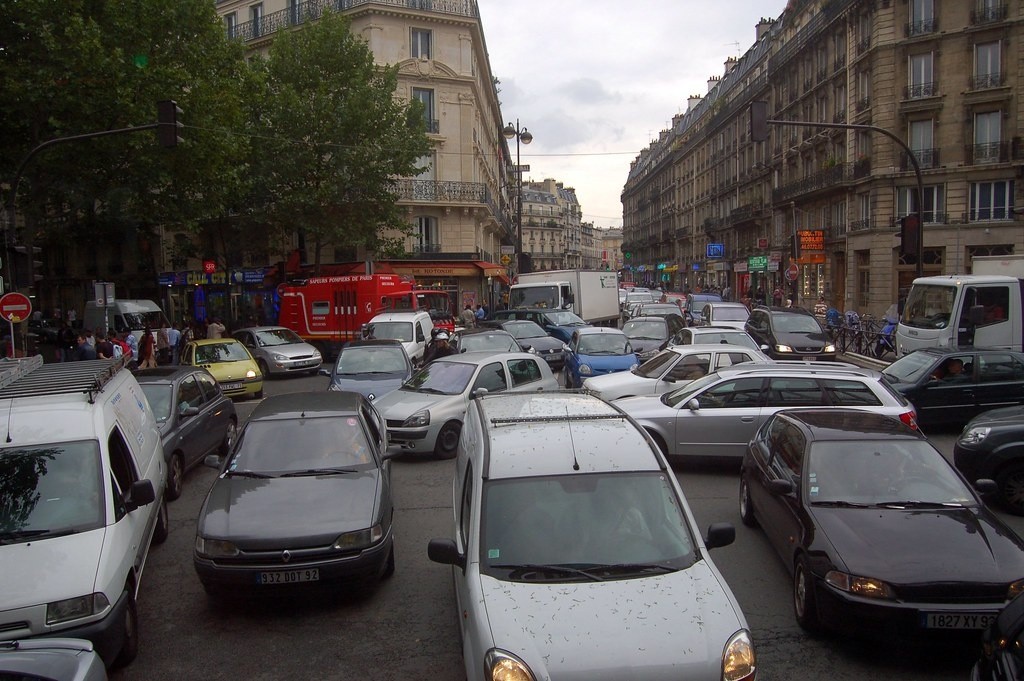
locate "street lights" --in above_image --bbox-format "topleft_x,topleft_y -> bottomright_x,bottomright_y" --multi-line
503,117 -> 533,273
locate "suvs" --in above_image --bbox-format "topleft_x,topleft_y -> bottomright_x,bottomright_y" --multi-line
607,359 -> 921,461
0,353 -> 167,681
426,378 -> 759,681
744,304 -> 837,363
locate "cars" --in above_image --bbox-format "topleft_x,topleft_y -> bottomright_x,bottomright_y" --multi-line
191,391 -> 403,601
560,327 -> 642,389
616,280 -> 773,364
965,589 -> 1024,681
737,406 -> 1024,632
580,343 -> 789,403
180,338 -> 263,399
444,307 -> 595,372
129,365 -> 238,500
954,404 -> 1024,519
368,351 -> 559,461
230,325 -> 324,379
883,343 -> 1024,428
319,339 -> 418,401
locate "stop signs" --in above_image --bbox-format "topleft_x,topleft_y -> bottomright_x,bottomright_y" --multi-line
788,265 -> 798,280
0,292 -> 33,322
278,283 -> 289,297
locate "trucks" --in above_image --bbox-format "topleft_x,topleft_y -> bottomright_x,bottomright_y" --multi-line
279,273 -> 456,364
507,269 -> 621,331
896,253 -> 1024,361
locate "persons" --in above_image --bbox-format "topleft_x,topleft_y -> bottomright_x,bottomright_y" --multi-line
979,296 -> 1004,325
462,299 -> 492,331
741,286 -> 765,308
680,354 -> 706,380
943,358 -> 967,385
422,327 -> 459,367
134,316 -> 226,370
813,297 -> 828,324
773,289 -> 783,307
496,290 -> 508,320
638,280 -> 692,298
695,283 -> 731,300
785,291 -> 796,309
0,307 -> 139,371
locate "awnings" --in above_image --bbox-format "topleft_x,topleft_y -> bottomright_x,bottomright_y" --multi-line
475,261 -> 506,276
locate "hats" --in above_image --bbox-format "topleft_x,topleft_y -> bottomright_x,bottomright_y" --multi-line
213,318 -> 221,323
119,328 -> 128,333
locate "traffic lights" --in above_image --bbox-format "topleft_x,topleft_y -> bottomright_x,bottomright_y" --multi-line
23,243 -> 45,288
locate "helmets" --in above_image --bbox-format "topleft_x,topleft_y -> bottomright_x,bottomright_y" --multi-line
436,332 -> 449,340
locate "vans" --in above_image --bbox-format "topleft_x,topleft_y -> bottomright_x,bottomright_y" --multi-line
83,298 -> 175,362
359,311 -> 436,367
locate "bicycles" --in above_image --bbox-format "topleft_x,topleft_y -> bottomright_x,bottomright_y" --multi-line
826,309 -> 900,360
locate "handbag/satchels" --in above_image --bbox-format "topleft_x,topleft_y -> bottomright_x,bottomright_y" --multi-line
154,351 -> 160,361
